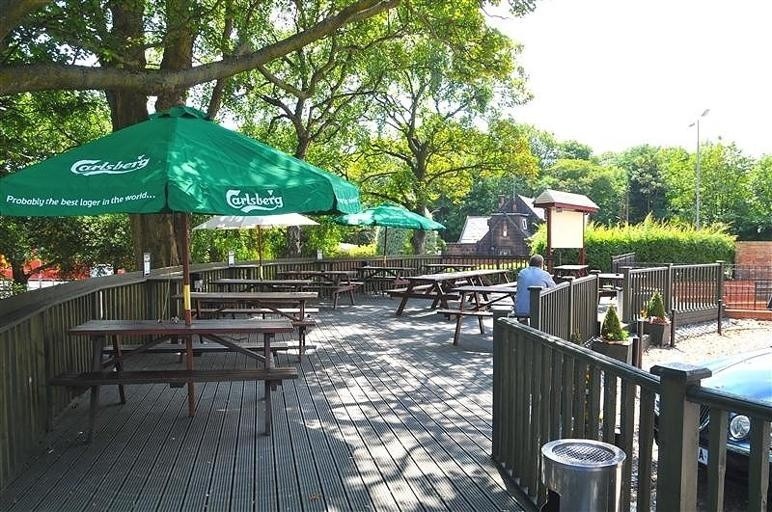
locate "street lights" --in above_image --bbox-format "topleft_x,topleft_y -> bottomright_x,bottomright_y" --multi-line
686,106 -> 713,233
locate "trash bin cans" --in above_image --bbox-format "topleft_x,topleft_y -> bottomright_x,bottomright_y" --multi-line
540,439 -> 626,511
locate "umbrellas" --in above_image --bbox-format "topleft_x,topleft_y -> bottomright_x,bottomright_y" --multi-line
189,209 -> 324,282
1,98 -> 363,416
331,199 -> 449,275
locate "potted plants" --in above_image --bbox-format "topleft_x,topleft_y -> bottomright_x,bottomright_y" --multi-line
639,291 -> 672,349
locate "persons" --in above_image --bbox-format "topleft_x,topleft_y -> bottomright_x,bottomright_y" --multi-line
513,253 -> 559,318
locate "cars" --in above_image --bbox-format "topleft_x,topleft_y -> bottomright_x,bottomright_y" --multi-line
655,351 -> 772,493
